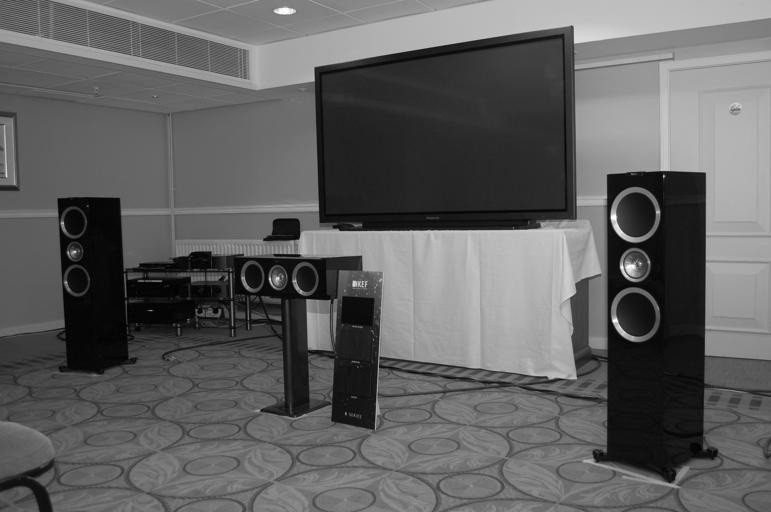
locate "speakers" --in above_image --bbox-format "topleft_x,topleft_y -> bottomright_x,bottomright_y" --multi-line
234,253 -> 364,300
56,197 -> 130,372
606,171 -> 707,471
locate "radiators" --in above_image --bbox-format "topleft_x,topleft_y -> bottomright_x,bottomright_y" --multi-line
173,236 -> 298,306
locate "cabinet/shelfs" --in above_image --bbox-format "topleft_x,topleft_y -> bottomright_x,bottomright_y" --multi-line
123,264 -> 251,338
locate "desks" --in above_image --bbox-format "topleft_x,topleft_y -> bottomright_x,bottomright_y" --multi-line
298,221 -> 591,382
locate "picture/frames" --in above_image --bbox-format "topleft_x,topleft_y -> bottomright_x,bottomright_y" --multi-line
0,109 -> 19,190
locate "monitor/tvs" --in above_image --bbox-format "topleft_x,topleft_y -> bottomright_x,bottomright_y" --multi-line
314,27 -> 577,232
